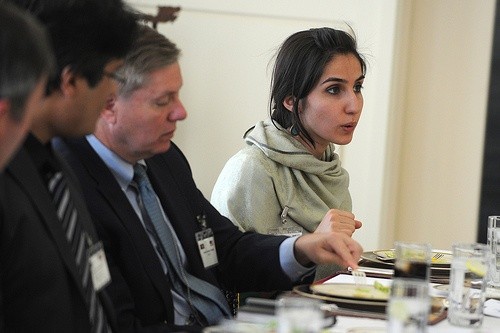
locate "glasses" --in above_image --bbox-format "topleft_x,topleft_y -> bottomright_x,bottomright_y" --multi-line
100,71 -> 128,95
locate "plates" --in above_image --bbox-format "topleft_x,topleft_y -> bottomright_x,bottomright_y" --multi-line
359,251 -> 450,271
293,284 -> 386,306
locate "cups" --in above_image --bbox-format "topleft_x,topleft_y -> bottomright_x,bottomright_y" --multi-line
386,242 -> 432,333
447,243 -> 489,328
274,294 -> 323,333
483,216 -> 500,318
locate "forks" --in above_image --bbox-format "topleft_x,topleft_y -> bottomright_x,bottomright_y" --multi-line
352,269 -> 366,277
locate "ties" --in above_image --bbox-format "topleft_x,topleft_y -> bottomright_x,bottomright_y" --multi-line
43,169 -> 113,333
133,162 -> 232,325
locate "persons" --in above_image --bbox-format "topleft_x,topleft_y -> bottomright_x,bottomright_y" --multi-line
210,21 -> 366,283
73,26 -> 363,333
0,0 -> 142,333
0,0 -> 54,173
